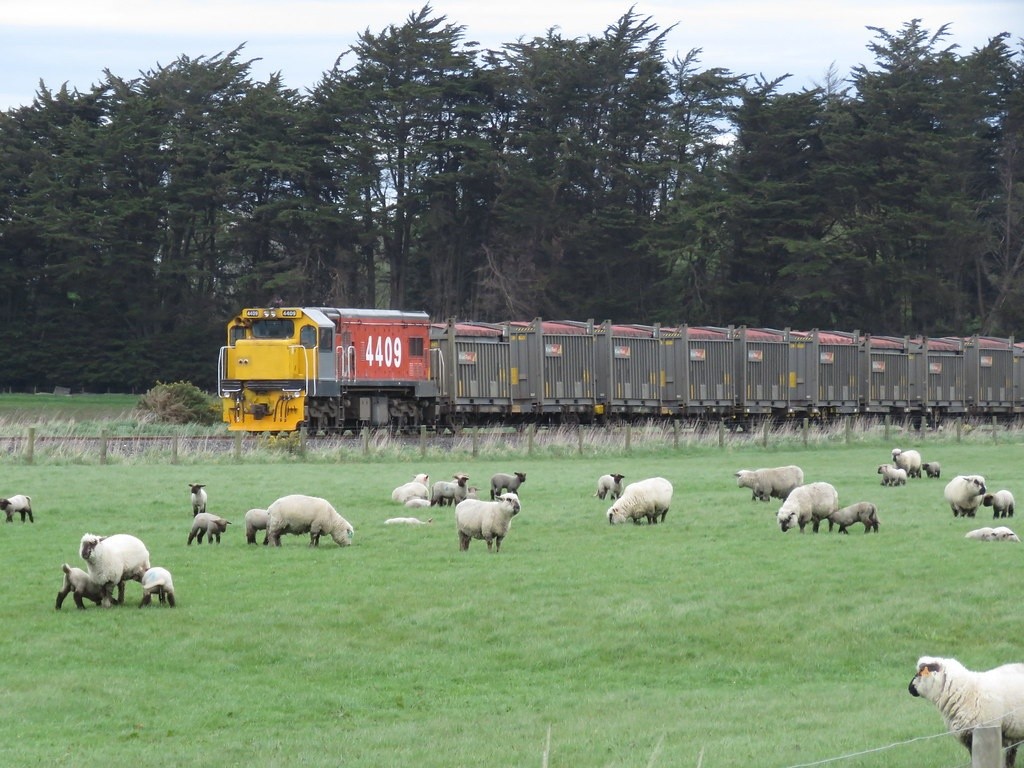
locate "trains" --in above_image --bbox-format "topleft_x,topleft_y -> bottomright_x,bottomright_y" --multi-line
218,308 -> 1024,435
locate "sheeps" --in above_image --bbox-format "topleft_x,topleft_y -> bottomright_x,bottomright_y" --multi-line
826,502 -> 879,534
594,473 -> 625,500
877,464 -> 907,486
188,483 -> 208,517
922,462 -> 940,479
944,474 -> 987,518
405,474 -> 482,508
245,508 -> 269,545
965,526 -> 1021,543
983,490 -> 1015,519
490,472 -> 526,500
892,448 -> 921,478
733,465 -> 803,502
391,473 -> 430,504
266,494 -> 354,548
187,512 -> 232,545
908,655 -> 1023,768
0,494 -> 34,523
55,533 -> 177,611
383,517 -> 433,525
606,477 -> 674,526
454,493 -> 521,553
776,481 -> 839,534
